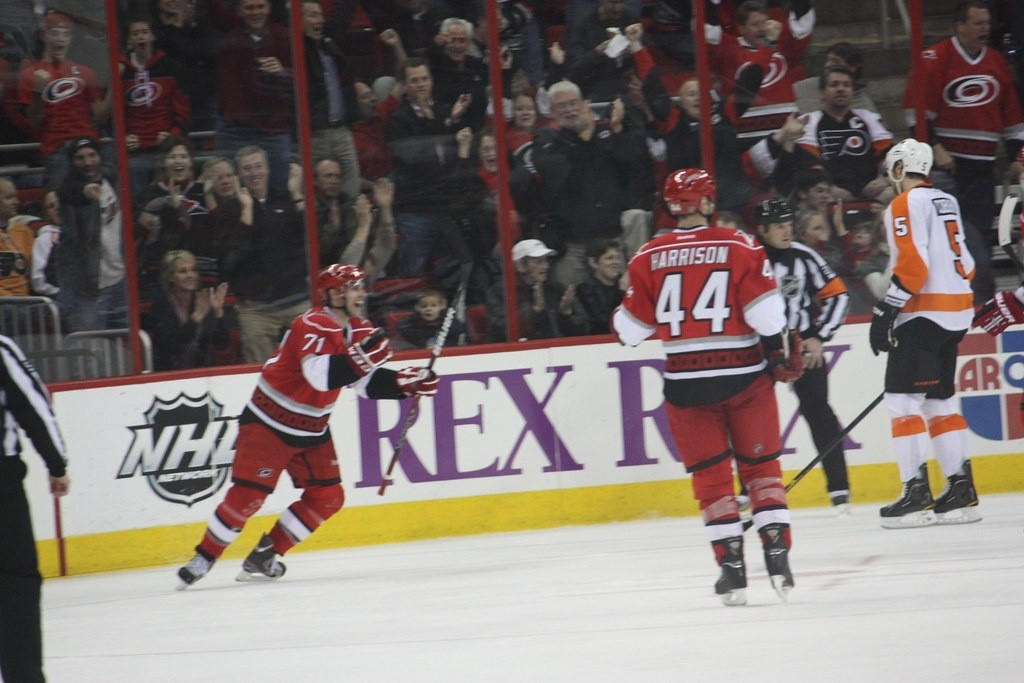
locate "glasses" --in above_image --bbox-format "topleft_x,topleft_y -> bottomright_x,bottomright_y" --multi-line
479,145 -> 497,153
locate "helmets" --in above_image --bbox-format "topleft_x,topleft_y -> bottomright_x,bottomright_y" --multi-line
1016,146 -> 1024,167
316,264 -> 365,300
754,199 -> 794,225
884,138 -> 934,183
663,168 -> 718,215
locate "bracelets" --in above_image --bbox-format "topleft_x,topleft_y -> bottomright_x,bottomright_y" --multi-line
293,198 -> 305,205
765,38 -> 777,45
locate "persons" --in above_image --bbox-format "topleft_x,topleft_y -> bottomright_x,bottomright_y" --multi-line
869,139 -> 979,517
0,335 -> 70,683
178,262 -> 440,584
611,168 -> 804,594
736,196 -> 851,507
0,0 -> 1024,371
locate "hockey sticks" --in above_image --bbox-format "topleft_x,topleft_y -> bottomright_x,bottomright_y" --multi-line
998,194 -> 1024,273
378,283 -> 463,495
740,391 -> 886,532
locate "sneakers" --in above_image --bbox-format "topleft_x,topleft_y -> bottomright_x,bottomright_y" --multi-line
704,517 -> 748,606
752,504 -> 795,603
175,544 -> 216,591
932,459 -> 983,525
826,483 -> 851,524
235,532 -> 286,583
879,462 -> 937,529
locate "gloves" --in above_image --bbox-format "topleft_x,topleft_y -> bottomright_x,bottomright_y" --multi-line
395,366 -> 440,398
345,327 -> 394,377
869,301 -> 901,357
970,290 -> 1024,338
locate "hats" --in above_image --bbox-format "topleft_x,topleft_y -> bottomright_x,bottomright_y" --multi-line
511,239 -> 557,261
69,135 -> 97,155
39,14 -> 72,35
